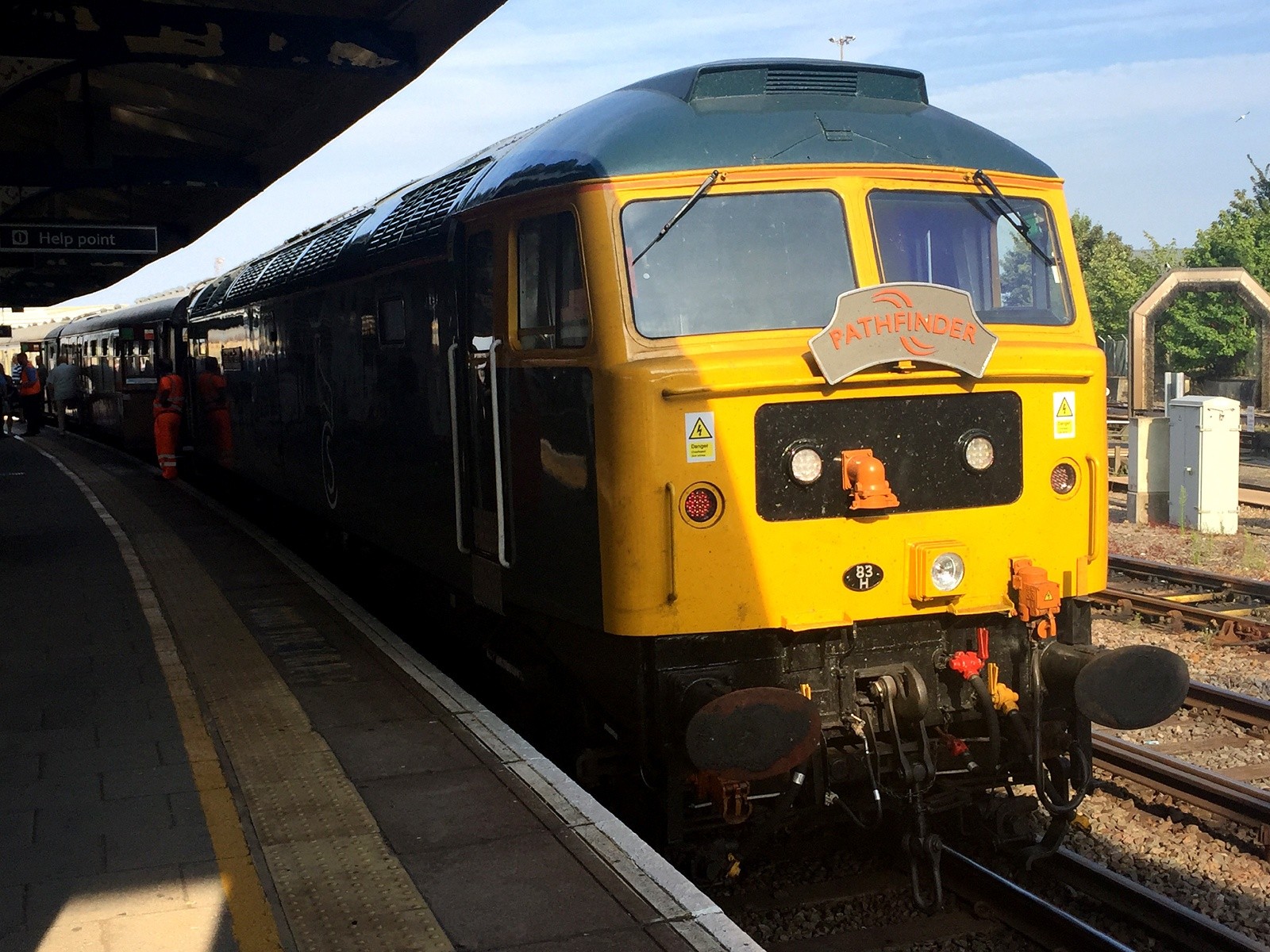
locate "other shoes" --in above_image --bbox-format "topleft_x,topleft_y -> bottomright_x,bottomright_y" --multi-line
153,474 -> 171,484
16,420 -> 27,424
56,432 -> 65,440
36,429 -> 41,434
21,431 -> 36,437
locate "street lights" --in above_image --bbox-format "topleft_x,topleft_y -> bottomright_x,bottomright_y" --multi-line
829,36 -> 857,61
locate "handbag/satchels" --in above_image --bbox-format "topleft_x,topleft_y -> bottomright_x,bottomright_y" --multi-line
3,401 -> 11,415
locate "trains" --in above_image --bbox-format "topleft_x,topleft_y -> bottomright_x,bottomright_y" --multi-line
0,55 -> 1192,916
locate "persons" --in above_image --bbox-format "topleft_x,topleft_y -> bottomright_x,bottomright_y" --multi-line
194,357 -> 234,471
82,357 -> 116,394
152,357 -> 184,480
0,353 -> 46,437
49,356 -> 77,435
